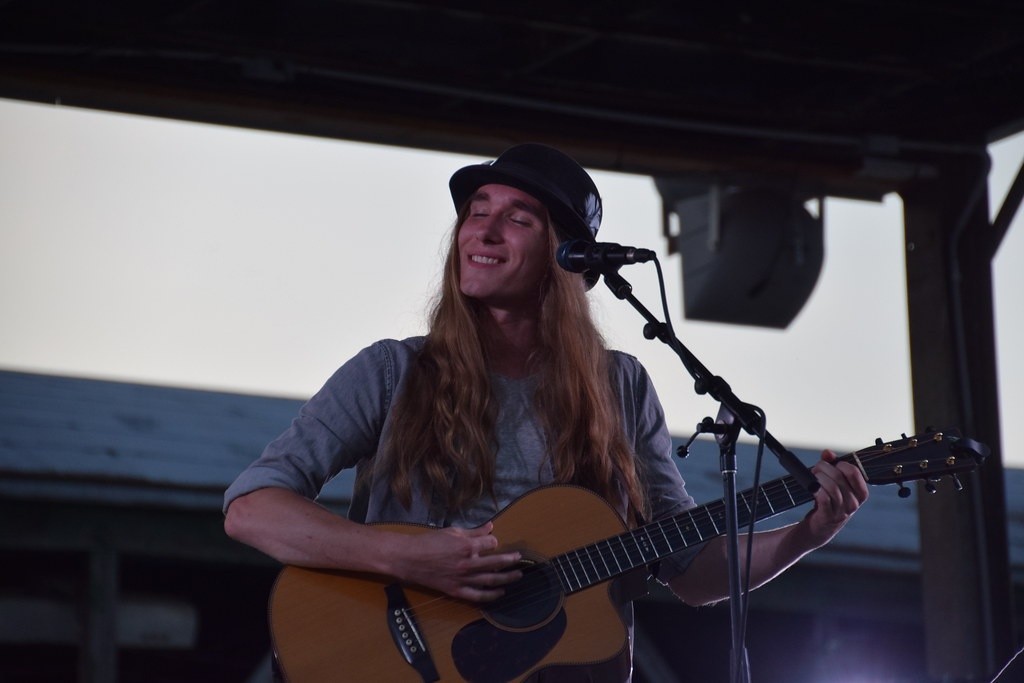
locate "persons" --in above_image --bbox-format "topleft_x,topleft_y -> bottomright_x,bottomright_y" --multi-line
220,140 -> 868,683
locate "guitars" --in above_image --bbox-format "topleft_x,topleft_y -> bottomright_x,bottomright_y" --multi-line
268,424 -> 992,682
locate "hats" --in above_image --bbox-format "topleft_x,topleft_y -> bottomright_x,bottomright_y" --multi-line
449,142 -> 604,293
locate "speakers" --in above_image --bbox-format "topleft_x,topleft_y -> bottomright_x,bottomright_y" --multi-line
675,184 -> 824,329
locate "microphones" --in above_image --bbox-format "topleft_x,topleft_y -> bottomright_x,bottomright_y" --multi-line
556,240 -> 655,274
